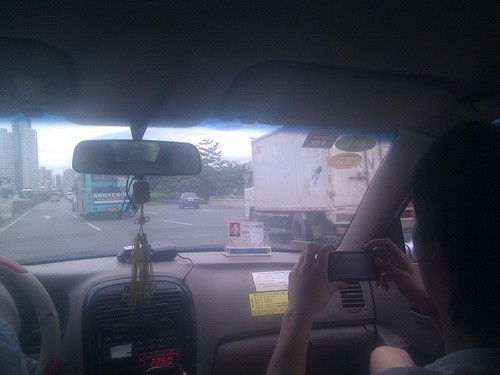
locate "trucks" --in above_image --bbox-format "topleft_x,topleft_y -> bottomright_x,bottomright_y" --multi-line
244,123 -> 415,245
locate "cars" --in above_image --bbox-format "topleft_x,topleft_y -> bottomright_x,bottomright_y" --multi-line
73,197 -> 78,211
70,196 -> 75,201
179,192 -> 200,210
50,195 -> 59,203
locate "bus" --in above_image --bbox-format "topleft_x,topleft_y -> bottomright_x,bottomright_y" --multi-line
19,187 -> 36,207
72,173 -> 135,219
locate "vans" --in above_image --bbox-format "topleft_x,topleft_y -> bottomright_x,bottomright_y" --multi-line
67,192 -> 74,200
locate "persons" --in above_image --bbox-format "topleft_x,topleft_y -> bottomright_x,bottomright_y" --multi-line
229,224 -> 241,237
266,121 -> 500,375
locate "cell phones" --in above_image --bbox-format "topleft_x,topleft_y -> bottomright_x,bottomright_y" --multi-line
328,250 -> 380,283
